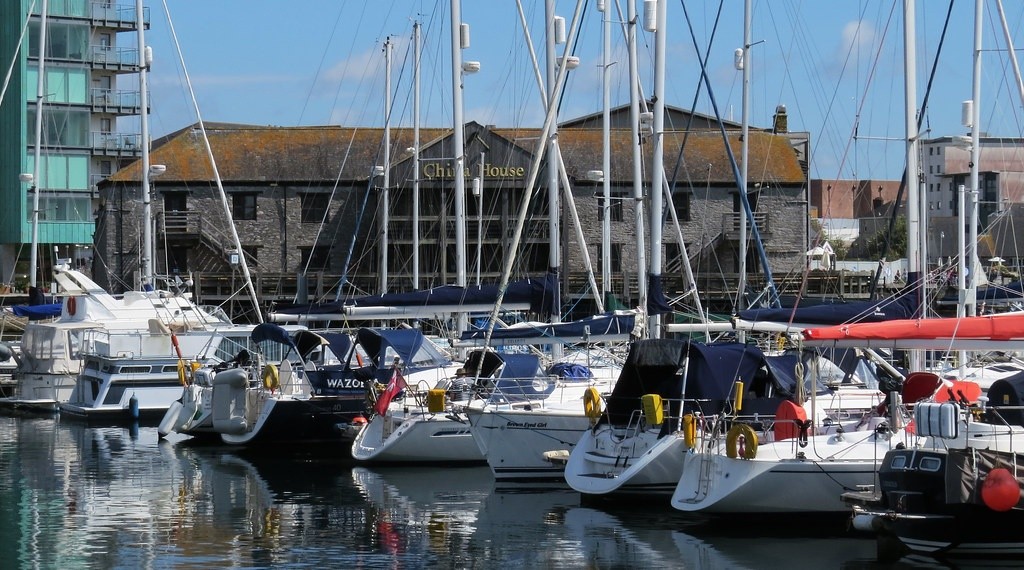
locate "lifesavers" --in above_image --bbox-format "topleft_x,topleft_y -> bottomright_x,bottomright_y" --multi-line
178,360 -> 185,384
584,388 -> 601,417
67,296 -> 76,315
263,364 -> 278,388
726,425 -> 758,458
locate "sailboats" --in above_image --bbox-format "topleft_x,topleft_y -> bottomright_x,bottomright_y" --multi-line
0,0 -> 1022,570
